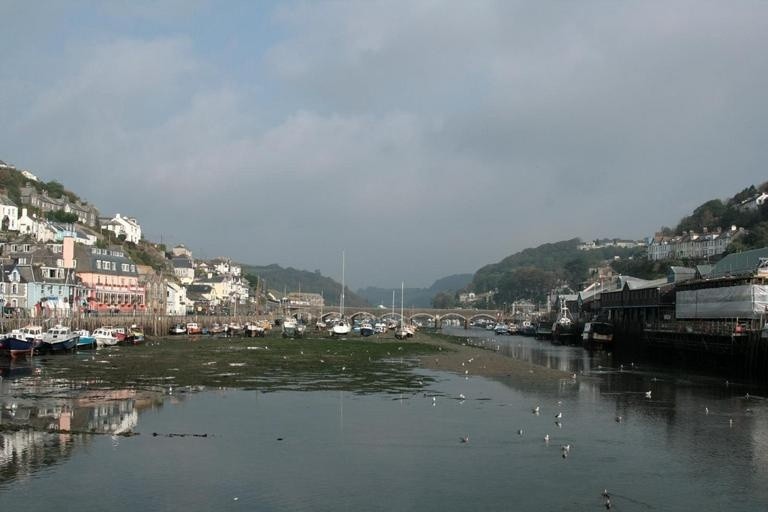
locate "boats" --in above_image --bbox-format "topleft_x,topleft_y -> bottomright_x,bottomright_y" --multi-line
169,251 -> 417,340
486,298 -> 614,350
0,324 -> 146,357
